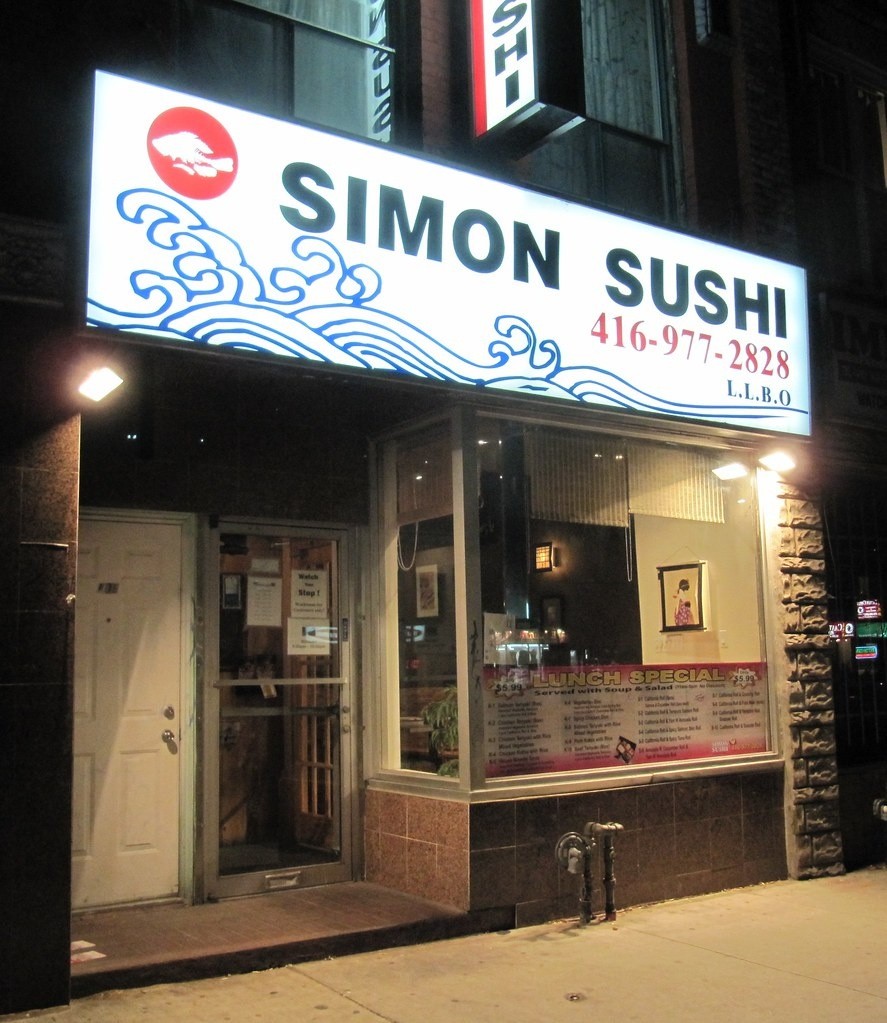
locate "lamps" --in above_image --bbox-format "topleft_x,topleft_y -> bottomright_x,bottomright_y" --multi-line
533,542 -> 558,573
